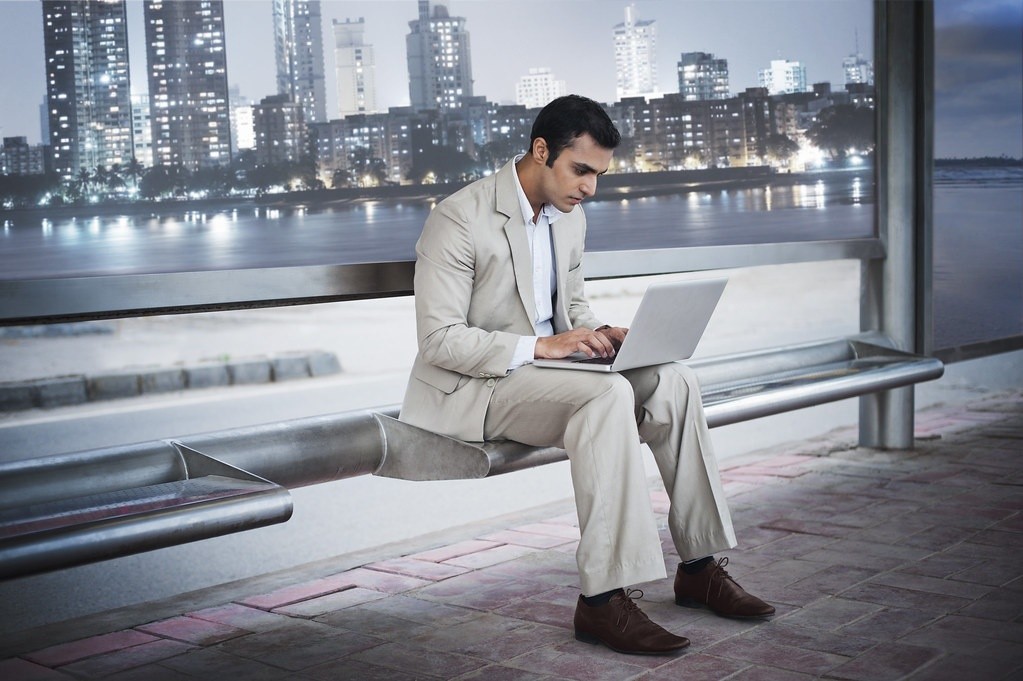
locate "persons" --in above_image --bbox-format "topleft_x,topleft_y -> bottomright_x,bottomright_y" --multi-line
397,94 -> 775,655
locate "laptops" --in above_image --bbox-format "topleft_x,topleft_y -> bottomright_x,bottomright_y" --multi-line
534,276 -> 729,373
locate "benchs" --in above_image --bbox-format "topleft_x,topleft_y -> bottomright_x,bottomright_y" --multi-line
0,336 -> 945,582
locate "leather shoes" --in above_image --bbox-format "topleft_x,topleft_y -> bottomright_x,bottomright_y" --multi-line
573,588 -> 691,655
674,557 -> 776,620
